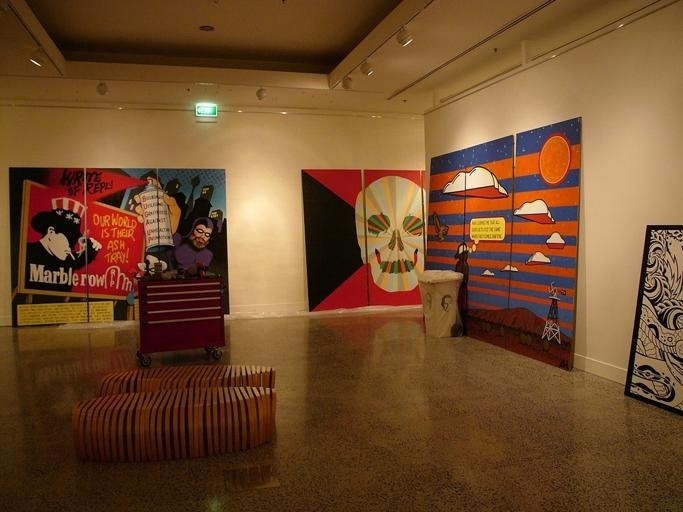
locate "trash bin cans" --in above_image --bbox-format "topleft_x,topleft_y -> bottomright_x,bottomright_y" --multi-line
417,269 -> 464,338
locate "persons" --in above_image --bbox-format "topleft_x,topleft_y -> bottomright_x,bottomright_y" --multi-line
24,207 -> 102,292
134,175 -> 214,280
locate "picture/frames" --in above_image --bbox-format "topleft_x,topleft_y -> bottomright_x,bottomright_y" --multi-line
624,224 -> 683,415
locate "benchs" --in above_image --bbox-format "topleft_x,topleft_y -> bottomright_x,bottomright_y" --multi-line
72,363 -> 277,462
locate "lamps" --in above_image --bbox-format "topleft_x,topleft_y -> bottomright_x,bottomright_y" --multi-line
255,88 -> 266,99
359,61 -> 374,78
396,26 -> 414,49
341,78 -> 352,90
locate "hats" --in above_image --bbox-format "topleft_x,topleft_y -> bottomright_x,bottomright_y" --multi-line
31,209 -> 82,243
